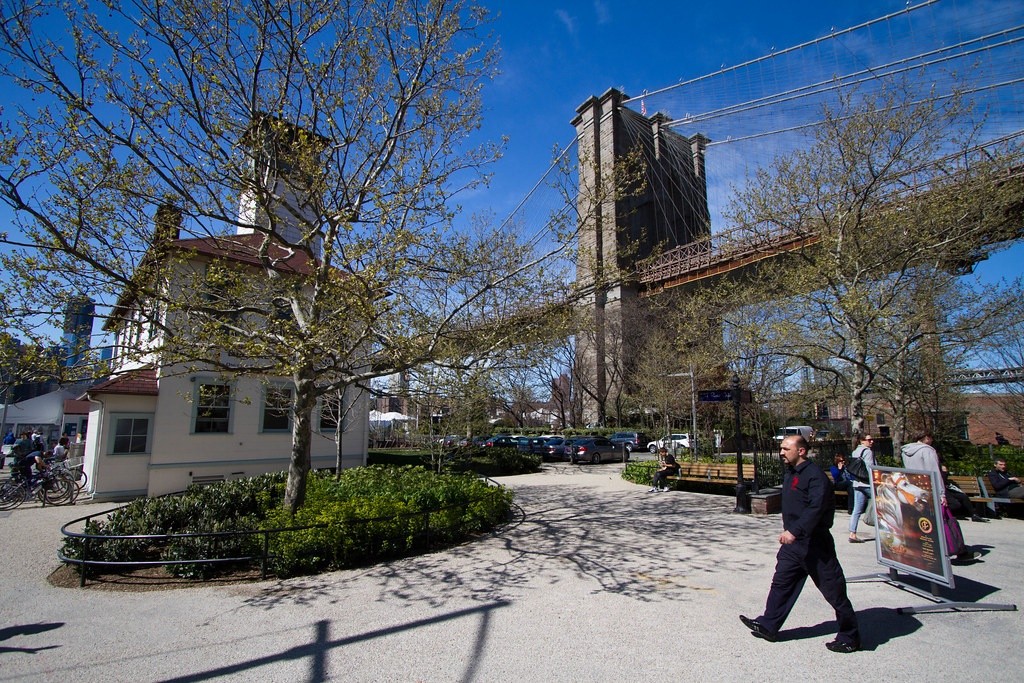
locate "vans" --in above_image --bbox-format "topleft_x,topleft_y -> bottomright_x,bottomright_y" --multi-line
772,426 -> 813,444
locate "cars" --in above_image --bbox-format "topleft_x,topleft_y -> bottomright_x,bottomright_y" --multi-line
563,437 -> 630,464
646,434 -> 700,454
609,431 -> 647,452
439,435 -> 578,462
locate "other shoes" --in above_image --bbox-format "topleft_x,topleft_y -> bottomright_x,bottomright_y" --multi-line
663,487 -> 669,492
648,486 -> 658,493
956,513 -> 969,520
849,537 -> 865,543
972,514 -> 990,522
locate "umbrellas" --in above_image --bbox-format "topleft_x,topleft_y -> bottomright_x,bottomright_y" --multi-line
369,410 -> 415,430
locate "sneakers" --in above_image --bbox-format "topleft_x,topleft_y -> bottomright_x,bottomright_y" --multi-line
740,615 -> 777,642
826,640 -> 864,653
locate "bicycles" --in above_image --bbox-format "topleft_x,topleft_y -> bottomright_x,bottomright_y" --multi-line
0,459 -> 88,511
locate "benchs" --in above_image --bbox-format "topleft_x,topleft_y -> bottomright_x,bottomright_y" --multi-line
657,461 -> 757,492
825,471 -> 1024,519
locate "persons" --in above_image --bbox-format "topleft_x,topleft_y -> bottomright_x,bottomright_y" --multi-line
19,451 -> 53,498
900,428 -> 982,561
53,437 -> 68,462
31,430 -> 38,441
849,433 -> 878,543
831,454 -> 854,515
739,435 -> 862,652
988,458 -> 1024,499
648,448 -> 677,492
35,432 -> 44,451
930,443 -> 987,522
62,432 -> 69,442
2,432 -> 8,441
3,432 -> 15,444
14,433 -> 33,482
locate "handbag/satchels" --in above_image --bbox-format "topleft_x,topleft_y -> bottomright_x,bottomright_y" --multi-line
863,498 -> 875,526
941,504 -> 964,555
836,481 -> 848,491
846,448 -> 875,484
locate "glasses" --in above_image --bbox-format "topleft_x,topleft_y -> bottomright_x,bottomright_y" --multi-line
839,459 -> 845,462
